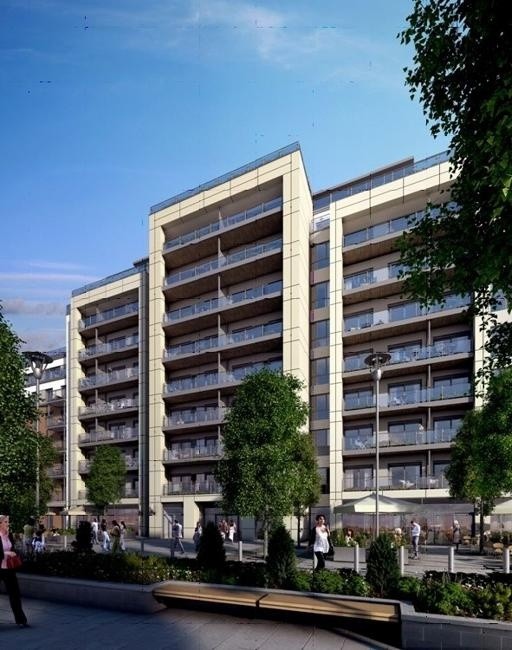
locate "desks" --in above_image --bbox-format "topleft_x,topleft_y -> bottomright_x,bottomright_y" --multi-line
461,538 -> 479,553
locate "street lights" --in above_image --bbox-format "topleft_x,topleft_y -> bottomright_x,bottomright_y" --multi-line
19,349 -> 54,532
363,350 -> 394,538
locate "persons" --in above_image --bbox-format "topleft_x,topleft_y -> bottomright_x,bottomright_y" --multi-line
170,519 -> 186,555
119,521 -> 126,552
193,518 -> 237,553
343,529 -> 354,547
409,520 -> 421,560
452,520 -> 462,553
12,518 -> 47,558
307,514 -> 331,573
108,519 -> 122,555
89,518 -> 101,546
0,514 -> 33,628
98,518 -> 113,553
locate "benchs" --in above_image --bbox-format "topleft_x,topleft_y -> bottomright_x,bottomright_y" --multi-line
259,592 -> 400,623
153,584 -> 268,607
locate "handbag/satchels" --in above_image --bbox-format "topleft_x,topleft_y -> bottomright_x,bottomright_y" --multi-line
323,537 -> 334,556
6,554 -> 22,569
